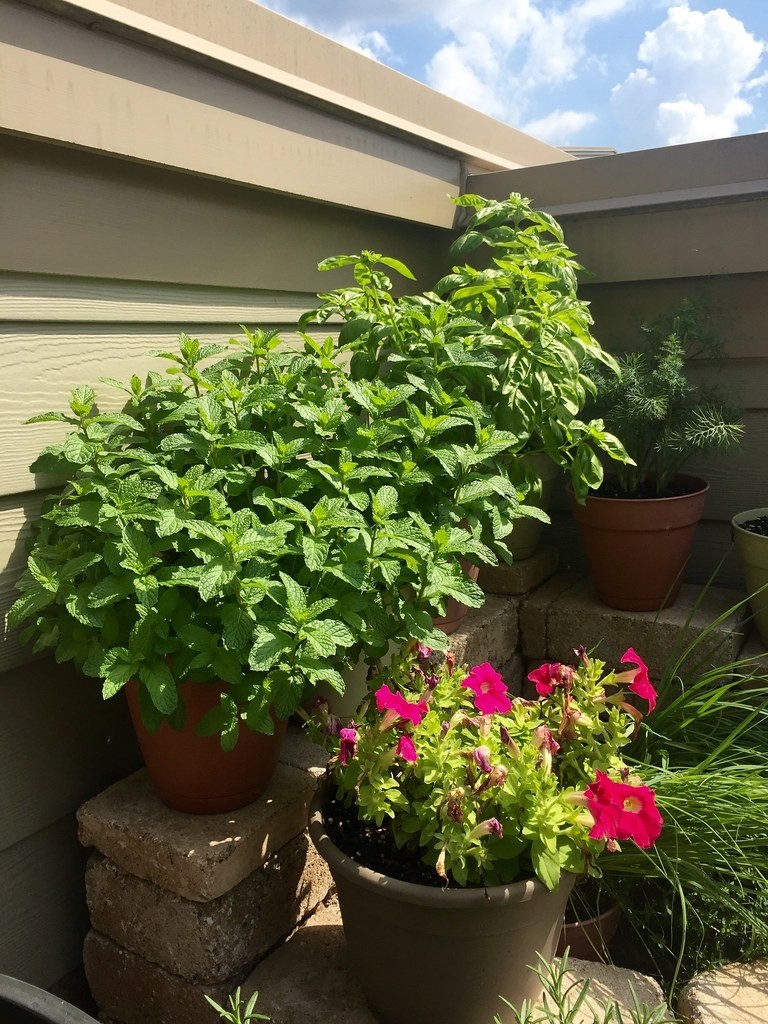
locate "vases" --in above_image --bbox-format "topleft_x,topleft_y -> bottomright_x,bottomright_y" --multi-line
732,505 -> 768,648
310,771 -> 585,1024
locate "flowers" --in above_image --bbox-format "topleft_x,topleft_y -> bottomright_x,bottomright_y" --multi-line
335,636 -> 663,885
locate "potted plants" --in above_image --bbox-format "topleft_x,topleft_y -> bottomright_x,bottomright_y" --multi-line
272,246 -> 630,640
4,324 -> 544,815
398,193 -> 623,559
554,554 -> 768,963
564,264 -> 748,614
